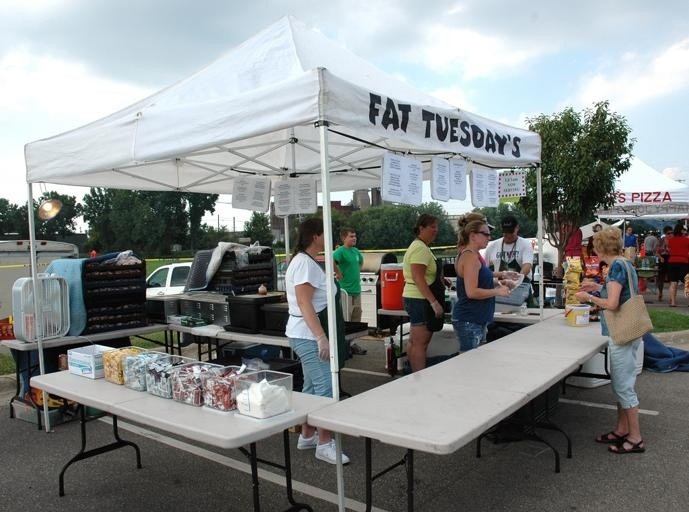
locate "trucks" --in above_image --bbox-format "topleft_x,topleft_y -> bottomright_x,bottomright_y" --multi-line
0,238 -> 81,321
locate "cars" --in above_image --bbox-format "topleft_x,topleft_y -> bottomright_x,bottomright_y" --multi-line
275,256 -> 325,270
144,261 -> 192,301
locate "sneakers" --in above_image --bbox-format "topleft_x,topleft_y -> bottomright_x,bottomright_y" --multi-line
351,343 -> 368,355
315,442 -> 351,465
482,427 -> 510,445
297,434 -> 335,451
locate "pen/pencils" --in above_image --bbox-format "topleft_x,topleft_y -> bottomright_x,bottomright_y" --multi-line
498,281 -> 504,288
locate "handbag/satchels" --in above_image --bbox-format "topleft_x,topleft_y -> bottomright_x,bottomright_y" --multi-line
603,295 -> 655,347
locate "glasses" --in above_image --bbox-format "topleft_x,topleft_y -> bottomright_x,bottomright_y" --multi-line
471,231 -> 492,236
667,232 -> 673,235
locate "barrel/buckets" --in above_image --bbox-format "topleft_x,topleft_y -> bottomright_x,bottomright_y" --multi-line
564,303 -> 592,328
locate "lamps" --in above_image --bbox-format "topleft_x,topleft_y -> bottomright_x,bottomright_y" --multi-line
36,181 -> 63,221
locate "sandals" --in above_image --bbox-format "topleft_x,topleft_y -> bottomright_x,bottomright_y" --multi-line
608,439 -> 646,455
596,431 -> 630,445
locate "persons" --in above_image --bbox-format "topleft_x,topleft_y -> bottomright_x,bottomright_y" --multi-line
586,218 -> 688,307
484,215 -> 534,308
574,226 -> 646,454
402,213 -> 445,373
332,227 -> 367,358
457,213 -> 521,281
451,213 -> 515,351
283,217 -> 351,466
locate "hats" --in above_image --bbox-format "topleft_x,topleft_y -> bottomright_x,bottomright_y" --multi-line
480,216 -> 495,232
500,217 -> 519,234
